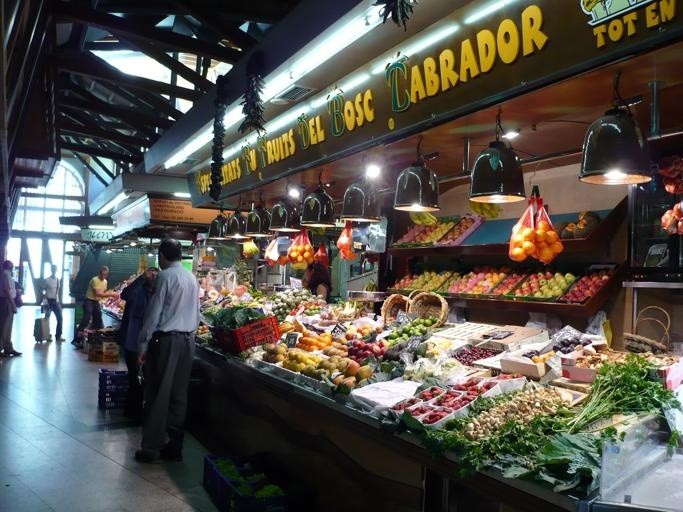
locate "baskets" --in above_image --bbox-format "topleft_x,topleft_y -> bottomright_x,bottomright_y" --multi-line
98,368 -> 129,409
88,330 -> 121,345
622,306 -> 671,354
380,289 -> 449,328
208,307 -> 282,355
203,453 -> 314,512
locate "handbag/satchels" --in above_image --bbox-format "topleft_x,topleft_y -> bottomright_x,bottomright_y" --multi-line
49,298 -> 56,306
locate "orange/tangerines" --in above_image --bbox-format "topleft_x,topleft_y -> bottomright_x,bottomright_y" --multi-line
387,317 -> 437,345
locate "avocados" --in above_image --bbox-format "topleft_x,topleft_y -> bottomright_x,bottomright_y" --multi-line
270,287 -> 326,322
383,341 -> 428,362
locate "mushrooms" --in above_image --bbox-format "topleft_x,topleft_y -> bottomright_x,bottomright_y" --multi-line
575,343 -> 678,369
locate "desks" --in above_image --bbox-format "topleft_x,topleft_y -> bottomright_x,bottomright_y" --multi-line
192,335 -> 669,512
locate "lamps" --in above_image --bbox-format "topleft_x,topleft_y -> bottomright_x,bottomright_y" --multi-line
208,201 -> 232,241
246,187 -> 274,237
88,221 -> 200,254
340,153 -> 381,223
97,190 -> 132,216
578,68 -> 653,185
467,106 -> 527,204
393,135 -> 441,212
299,168 -> 337,228
268,176 -> 300,233
201,1 -> 512,165
162,2 -> 409,170
225,195 -> 251,239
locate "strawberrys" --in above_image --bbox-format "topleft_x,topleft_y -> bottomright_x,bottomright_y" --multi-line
394,372 -> 523,426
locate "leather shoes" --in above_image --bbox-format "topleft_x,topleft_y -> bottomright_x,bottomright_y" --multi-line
3,351 -> 22,355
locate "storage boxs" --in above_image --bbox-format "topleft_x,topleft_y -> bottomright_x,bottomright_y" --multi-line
82,332 -> 121,362
387,265 -> 617,304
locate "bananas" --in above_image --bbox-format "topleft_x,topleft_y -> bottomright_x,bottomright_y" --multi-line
241,242 -> 259,260
364,281 -> 378,291
469,201 -> 504,219
278,321 -> 297,336
409,212 -> 437,226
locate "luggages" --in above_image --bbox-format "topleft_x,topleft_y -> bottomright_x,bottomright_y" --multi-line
34,296 -> 50,343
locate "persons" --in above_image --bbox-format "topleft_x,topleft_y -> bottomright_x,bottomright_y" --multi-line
133,237 -> 201,465
305,259 -> 334,306
119,266 -> 161,426
37,264 -> 65,344
0,258 -> 23,359
71,265 -> 115,347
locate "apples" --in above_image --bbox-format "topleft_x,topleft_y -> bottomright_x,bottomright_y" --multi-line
397,218 -> 475,245
263,342 -> 289,363
394,272 -> 612,305
297,325 -> 385,380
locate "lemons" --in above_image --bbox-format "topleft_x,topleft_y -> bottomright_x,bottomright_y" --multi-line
283,351 -> 324,372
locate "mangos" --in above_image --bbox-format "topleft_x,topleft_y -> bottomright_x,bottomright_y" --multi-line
332,360 -> 372,389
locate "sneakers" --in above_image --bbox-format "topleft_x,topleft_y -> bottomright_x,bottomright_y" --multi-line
134,450 -> 183,464
47,338 -> 65,342
71,341 -> 83,349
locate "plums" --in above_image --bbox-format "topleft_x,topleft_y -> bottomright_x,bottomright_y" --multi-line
522,338 -> 592,359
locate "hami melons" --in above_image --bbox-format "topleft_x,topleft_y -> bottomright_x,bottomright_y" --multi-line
553,211 -> 599,238
